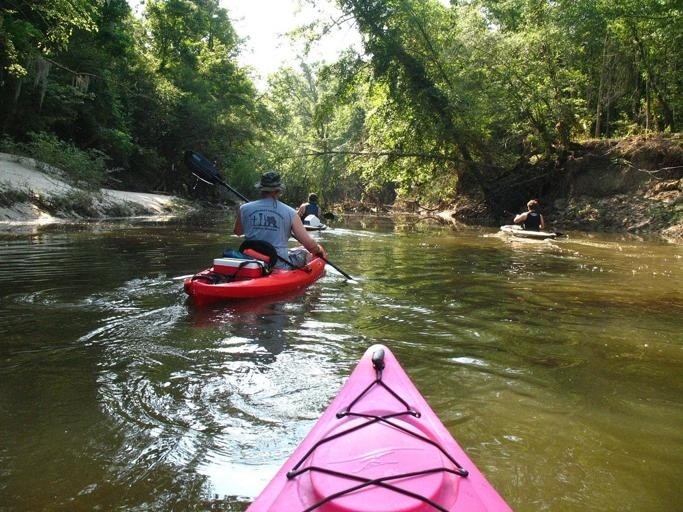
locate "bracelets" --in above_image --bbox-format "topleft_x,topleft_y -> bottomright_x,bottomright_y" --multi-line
315,245 -> 320,253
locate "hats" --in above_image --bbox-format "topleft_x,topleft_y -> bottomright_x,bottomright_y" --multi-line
255,172 -> 286,192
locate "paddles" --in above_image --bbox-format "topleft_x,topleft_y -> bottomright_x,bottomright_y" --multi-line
183,147 -> 353,279
320,212 -> 334,220
494,203 -> 564,237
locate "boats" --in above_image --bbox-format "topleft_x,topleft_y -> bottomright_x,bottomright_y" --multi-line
499,224 -> 560,239
302,214 -> 327,230
183,244 -> 328,306
241,344 -> 514,511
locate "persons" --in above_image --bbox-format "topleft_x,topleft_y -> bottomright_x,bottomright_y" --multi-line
232,171 -> 327,274
295,192 -> 322,223
513,200 -> 545,232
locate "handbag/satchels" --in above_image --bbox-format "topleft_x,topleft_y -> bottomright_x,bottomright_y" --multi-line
239,239 -> 277,267
